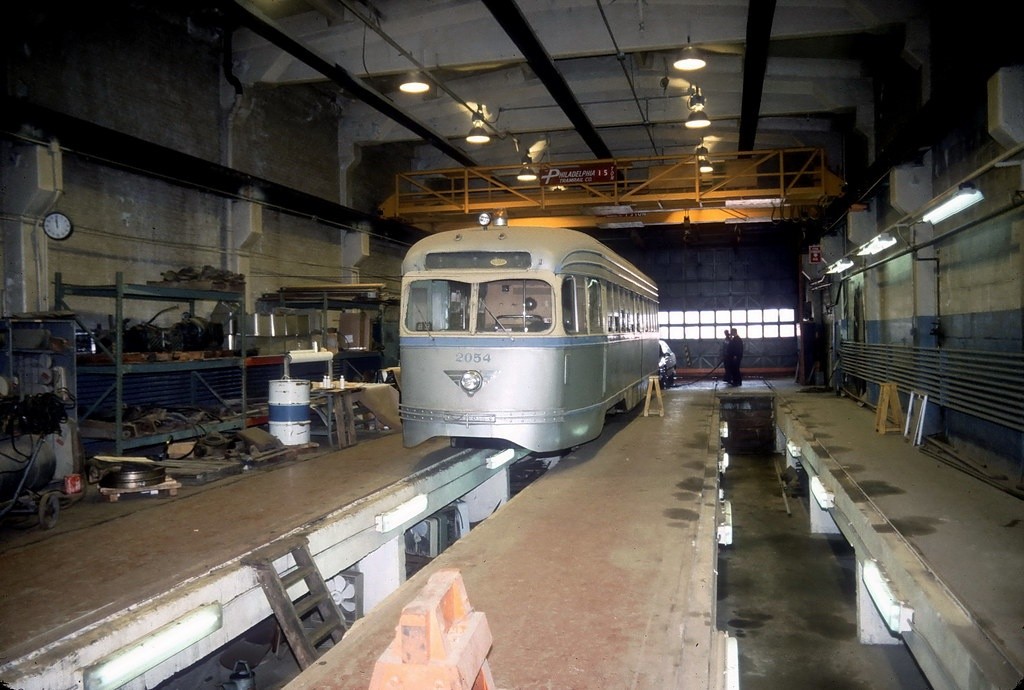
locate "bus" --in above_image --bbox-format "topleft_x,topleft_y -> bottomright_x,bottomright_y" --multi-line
398,208 -> 659,454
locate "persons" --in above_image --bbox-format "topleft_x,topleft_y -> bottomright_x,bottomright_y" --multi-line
720,327 -> 744,386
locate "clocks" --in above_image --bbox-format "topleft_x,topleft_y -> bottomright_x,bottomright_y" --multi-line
42,211 -> 74,242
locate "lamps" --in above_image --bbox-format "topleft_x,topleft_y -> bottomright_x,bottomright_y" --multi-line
717,500 -> 733,546
465,104 -> 490,144
724,630 -> 739,690
697,136 -> 713,173
922,181 -> 984,226
786,438 -> 802,458
718,448 -> 729,473
862,558 -> 913,635
516,149 -> 537,181
720,421 -> 729,438
856,233 -> 897,256
684,86 -> 712,128
672,36 -> 707,71
812,475 -> 835,510
399,50 -> 430,93
826,257 -> 855,274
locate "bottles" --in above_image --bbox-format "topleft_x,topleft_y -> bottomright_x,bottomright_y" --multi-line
326,376 -> 331,389
323,375 -> 327,388
339,375 -> 346,388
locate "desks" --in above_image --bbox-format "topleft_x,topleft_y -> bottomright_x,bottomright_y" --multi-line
309,379 -> 390,446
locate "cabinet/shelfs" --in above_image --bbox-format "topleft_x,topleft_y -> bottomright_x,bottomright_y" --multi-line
51,271 -> 248,458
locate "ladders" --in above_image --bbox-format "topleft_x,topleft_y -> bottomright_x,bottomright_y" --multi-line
874,382 -> 905,435
643,376 -> 664,417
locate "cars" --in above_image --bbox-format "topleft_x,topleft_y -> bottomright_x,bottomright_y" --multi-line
659,340 -> 677,390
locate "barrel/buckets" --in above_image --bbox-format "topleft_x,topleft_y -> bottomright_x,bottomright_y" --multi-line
267,380 -> 311,445
